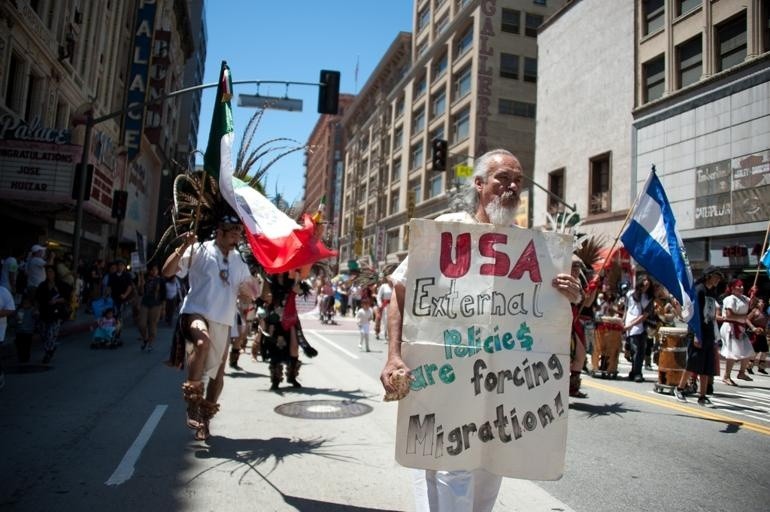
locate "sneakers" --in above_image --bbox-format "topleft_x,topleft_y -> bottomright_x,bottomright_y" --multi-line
673,385 -> 688,403
141,340 -> 155,352
697,395 -> 717,409
628,370 -> 645,382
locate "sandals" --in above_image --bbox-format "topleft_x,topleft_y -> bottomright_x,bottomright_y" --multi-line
182,380 -> 220,442
722,376 -> 737,386
737,370 -> 753,381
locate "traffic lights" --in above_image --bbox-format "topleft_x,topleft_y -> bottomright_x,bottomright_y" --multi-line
317,72 -> 341,113
432,139 -> 448,171
110,191 -> 126,217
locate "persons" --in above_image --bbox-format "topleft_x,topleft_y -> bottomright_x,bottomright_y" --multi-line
570,259 -> 769,407
378,149 -> 583,511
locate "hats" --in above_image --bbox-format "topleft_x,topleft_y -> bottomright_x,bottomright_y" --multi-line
31,244 -> 47,254
215,212 -> 246,233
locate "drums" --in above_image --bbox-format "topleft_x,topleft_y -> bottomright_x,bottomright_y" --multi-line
657,327 -> 689,387
592,316 -> 624,373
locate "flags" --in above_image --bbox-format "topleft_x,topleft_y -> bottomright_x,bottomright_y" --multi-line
760,246 -> 770,270
205,64 -> 339,270
620,172 -> 701,342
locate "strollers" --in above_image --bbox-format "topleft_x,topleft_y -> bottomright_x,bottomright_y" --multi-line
89,295 -> 123,349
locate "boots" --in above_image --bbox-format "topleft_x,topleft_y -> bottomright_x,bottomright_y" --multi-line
268,361 -> 282,391
756,358 -> 770,376
229,349 -> 244,371
286,358 -> 303,389
746,359 -> 756,375
569,370 -> 586,398
